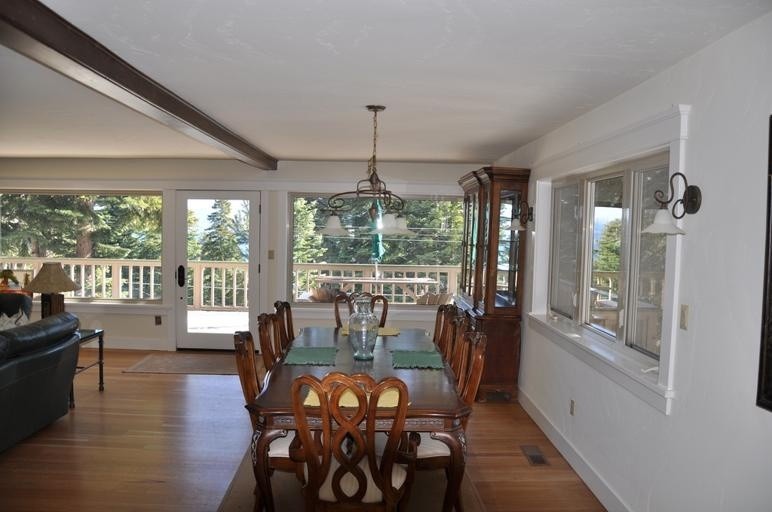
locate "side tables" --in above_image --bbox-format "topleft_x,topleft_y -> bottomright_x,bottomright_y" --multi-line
69,324 -> 105,409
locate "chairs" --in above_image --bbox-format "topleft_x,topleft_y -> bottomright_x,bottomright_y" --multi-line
373,303 -> 487,512
233,300 -> 324,512
293,373 -> 422,511
334,292 -> 389,329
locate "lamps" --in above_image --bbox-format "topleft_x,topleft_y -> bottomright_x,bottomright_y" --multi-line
24,262 -> 81,319
509,200 -> 532,233
315,105 -> 418,237
639,171 -> 702,237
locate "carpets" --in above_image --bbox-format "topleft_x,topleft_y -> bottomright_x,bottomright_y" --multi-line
121,351 -> 265,375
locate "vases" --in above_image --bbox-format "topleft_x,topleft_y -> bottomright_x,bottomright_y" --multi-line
349,298 -> 379,360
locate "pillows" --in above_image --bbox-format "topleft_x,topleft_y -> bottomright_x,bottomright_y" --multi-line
0,311 -> 28,331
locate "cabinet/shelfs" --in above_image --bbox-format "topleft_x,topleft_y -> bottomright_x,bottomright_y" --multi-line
453,167 -> 530,404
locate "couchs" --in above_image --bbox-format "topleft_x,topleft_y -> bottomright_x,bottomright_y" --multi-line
0,310 -> 81,459
0,292 -> 32,319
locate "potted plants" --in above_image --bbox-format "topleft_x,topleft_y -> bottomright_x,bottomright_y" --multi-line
0,268 -> 18,290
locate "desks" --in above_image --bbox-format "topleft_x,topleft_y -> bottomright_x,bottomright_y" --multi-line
245,326 -> 472,512
